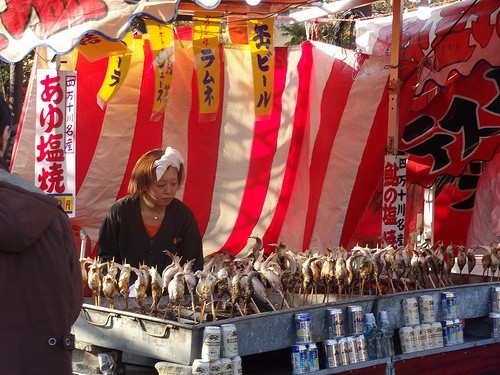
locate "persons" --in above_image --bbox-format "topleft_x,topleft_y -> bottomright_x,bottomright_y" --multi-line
0,97 -> 84,375
96,146 -> 204,296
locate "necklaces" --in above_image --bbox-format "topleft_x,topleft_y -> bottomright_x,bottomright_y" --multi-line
149,208 -> 164,220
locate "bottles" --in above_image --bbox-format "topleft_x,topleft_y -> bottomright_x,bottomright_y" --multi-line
379,311 -> 395,358
363,313 -> 384,361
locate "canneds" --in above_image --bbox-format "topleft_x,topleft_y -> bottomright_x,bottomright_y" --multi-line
201,326 -> 221,359
402,297 -> 420,326
291,344 -> 319,374
324,305 -> 369,368
440,292 -> 464,347
399,326 -> 416,354
431,322 -> 444,348
220,324 -> 238,357
419,295 -> 434,323
420,323 -> 433,349
191,354 -> 243,375
294,313 -> 313,343
489,287 -> 500,338
412,324 -> 424,351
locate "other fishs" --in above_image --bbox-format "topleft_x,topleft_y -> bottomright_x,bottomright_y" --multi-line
78,237 -> 500,305
